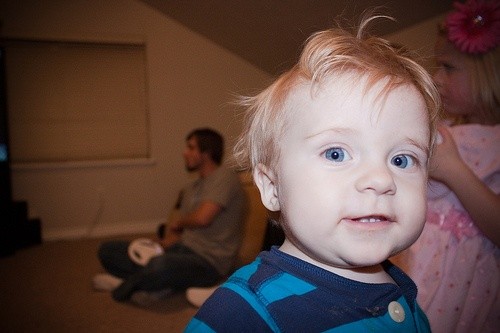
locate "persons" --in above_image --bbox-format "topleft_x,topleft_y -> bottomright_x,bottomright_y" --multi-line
91,128 -> 249,306
181,15 -> 443,333
401,0 -> 500,333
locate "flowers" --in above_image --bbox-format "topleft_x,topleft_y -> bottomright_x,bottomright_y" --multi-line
444,0 -> 500,55
